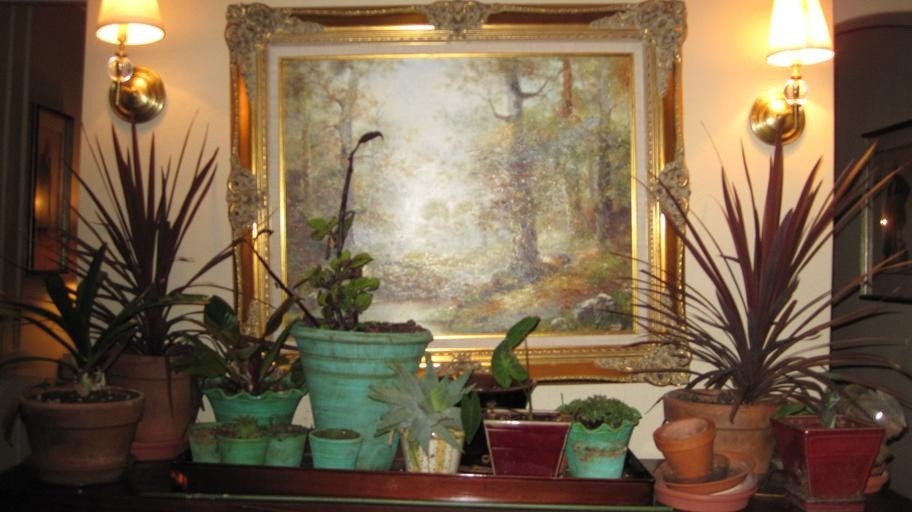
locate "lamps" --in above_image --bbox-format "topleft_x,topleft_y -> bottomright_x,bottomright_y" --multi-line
94,1 -> 171,122
750,0 -> 835,145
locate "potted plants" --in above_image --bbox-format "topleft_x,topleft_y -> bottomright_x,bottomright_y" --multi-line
614,115 -> 907,493
480,335 -> 573,477
429,312 -> 540,460
368,355 -> 479,474
556,396 -> 641,477
1,113 -> 432,511
769,370 -> 887,512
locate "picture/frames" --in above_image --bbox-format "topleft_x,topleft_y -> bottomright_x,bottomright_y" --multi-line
223,0 -> 693,390
